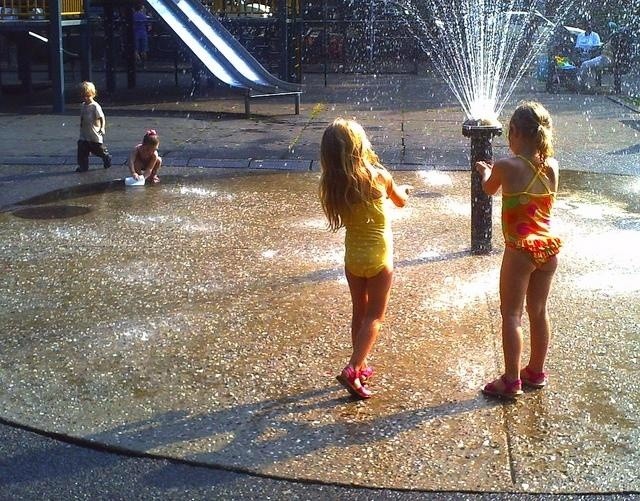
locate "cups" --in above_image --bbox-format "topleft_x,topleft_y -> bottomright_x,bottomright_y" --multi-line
125,175 -> 145,186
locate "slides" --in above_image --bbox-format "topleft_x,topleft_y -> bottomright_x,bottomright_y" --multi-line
143,0 -> 306,118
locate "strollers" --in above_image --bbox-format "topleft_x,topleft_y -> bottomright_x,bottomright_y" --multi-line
541,22 -> 586,96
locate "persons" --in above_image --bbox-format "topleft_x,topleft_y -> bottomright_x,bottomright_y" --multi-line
317,118 -> 410,399
75,81 -> 112,172
132,3 -> 152,61
476,102 -> 564,397
575,20 -> 631,86
128,130 -> 162,183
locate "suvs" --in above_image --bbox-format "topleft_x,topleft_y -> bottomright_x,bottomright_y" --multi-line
492,2 -> 601,40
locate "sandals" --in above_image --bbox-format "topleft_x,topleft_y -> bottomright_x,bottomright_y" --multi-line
336,364 -> 373,399
76,167 -> 89,171
152,175 -> 160,184
481,366 -> 547,398
104,154 -> 113,169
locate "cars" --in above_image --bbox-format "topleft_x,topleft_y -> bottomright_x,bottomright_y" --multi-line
154,0 -> 349,67
